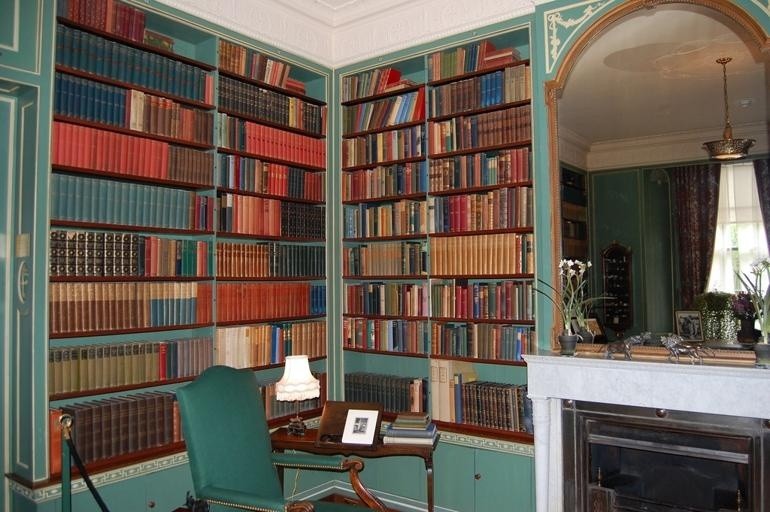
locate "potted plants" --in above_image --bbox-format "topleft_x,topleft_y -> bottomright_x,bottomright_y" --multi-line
692,291 -> 739,345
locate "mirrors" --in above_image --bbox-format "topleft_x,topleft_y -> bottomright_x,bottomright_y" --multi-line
544,0 -> 770,359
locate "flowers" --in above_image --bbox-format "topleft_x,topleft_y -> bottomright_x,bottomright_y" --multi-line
730,291 -> 764,320
732,252 -> 770,343
561,260 -> 616,326
534,259 -> 620,334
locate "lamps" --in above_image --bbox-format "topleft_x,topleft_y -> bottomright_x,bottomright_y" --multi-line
275,356 -> 321,436
703,56 -> 756,160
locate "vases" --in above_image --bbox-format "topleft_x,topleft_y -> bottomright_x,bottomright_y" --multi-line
579,330 -> 596,345
558,334 -> 577,356
736,317 -> 762,346
755,344 -> 769,367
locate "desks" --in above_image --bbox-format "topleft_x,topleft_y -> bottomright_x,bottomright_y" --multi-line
270,427 -> 441,512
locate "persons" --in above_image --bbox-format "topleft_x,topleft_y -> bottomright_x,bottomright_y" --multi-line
682,316 -> 698,337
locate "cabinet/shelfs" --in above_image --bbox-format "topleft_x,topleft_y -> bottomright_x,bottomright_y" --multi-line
47,0 -> 550,511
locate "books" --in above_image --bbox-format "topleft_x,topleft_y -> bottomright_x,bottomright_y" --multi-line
344,187 -> 532,239
561,219 -> 587,262
343,318 -> 535,362
218,320 -> 326,370
261,373 -> 326,421
383,412 -> 438,445
57,25 -> 213,146
342,39 -> 529,133
343,105 -> 530,167
343,234 -> 533,275
219,154 -> 326,239
218,39 -> 306,95
342,148 -> 533,200
431,359 -> 534,434
344,371 -> 429,414
50,121 -> 213,232
344,278 -> 534,320
49,231 -> 213,333
220,76 -> 325,168
216,246 -> 326,322
58,1 -> 145,42
50,390 -> 185,474
49,337 -> 213,395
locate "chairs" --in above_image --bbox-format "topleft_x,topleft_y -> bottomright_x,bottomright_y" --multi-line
176,365 -> 388,512
